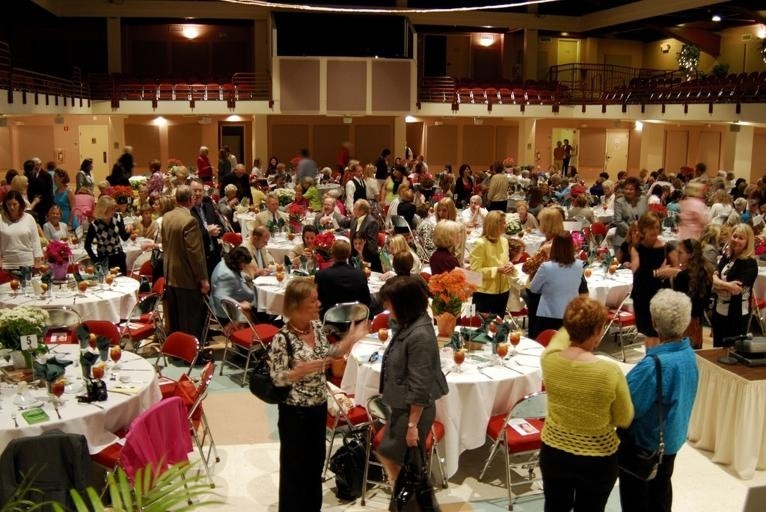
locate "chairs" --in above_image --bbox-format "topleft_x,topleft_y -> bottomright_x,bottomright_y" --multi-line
88,396 -> 192,512
326,383 -> 379,482
478,391 -> 548,511
187,361 -> 220,488
361,393 -> 448,506
14,437 -> 72,512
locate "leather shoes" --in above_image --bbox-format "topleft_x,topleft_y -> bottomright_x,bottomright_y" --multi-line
185,357 -> 214,365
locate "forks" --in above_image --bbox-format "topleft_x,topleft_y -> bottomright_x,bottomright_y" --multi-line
12,413 -> 20,427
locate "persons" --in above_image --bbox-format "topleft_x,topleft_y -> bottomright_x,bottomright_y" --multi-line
267,281 -> 368,512
539,295 -> 635,512
0,139 -> 765,351
373,276 -> 449,512
614,289 -> 699,512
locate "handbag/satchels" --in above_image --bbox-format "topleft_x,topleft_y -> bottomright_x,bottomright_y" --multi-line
248,332 -> 293,404
327,382 -> 353,417
329,429 -> 382,500
388,438 -> 441,512
151,248 -> 164,271
615,428 -> 665,482
123,334 -> 158,355
172,373 -> 202,430
737,312 -> 766,337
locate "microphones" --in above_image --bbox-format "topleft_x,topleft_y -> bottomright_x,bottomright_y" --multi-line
722,333 -> 753,342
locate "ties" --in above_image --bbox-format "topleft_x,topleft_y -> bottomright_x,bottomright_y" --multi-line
272,212 -> 277,223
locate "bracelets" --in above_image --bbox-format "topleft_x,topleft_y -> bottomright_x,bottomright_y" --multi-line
407,421 -> 418,430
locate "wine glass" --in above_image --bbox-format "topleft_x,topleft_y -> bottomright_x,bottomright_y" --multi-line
93,367 -> 104,381
61,235 -> 80,249
497,344 -> 508,367
51,382 -> 65,395
585,260 -> 618,279
288,233 -> 294,246
518,230 -> 524,239
10,265 -> 119,297
602,204 -> 608,212
111,348 -> 121,369
379,328 -> 389,344
89,336 -> 98,351
276,264 -> 286,286
130,232 -> 138,244
364,267 -> 371,280
455,349 -> 465,374
510,333 -> 520,355
